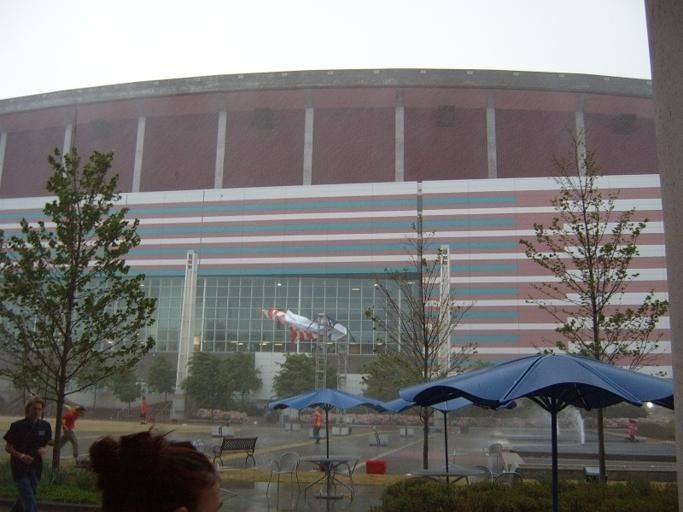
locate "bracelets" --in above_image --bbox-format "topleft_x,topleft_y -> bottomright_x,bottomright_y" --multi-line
19,454 -> 24,459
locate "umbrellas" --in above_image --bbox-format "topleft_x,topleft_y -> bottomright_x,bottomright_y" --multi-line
376,397 -> 517,484
398,353 -> 673,512
268,388 -> 385,459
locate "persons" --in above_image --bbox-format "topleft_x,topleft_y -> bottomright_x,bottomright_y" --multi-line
61,407 -> 86,458
3,395 -> 52,512
89,431 -> 224,512
626,420 -> 636,441
312,409 -> 321,444
140,397 -> 147,424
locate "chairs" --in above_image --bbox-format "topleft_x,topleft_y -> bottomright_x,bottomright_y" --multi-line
466,465 -> 493,486
495,472 -> 522,490
267,453 -> 300,500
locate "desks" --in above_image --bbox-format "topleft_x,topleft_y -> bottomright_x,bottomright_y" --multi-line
414,470 -> 471,486
297,457 -> 355,512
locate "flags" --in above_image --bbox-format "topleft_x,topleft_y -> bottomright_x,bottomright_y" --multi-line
262,307 -> 347,344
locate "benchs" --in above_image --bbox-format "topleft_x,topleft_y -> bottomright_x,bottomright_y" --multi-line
213,437 -> 257,467
